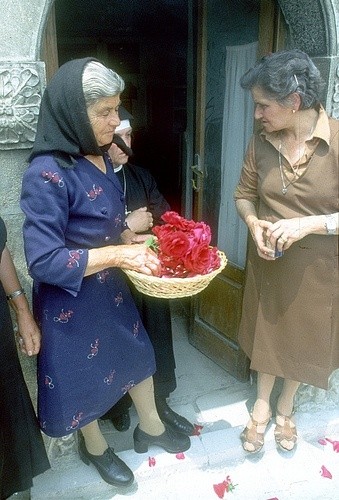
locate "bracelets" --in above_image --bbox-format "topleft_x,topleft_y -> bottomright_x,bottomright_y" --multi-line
6,288 -> 24,301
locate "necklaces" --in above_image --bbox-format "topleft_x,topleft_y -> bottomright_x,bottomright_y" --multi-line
279,120 -> 315,194
122,168 -> 126,196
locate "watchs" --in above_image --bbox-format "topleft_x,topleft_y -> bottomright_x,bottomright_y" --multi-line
325,214 -> 337,234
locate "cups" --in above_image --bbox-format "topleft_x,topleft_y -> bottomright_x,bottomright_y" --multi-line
263,227 -> 285,258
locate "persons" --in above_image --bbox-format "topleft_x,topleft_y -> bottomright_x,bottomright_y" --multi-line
100,107 -> 194,435
19,58 -> 190,487
0,217 -> 51,500
233,50 -> 339,453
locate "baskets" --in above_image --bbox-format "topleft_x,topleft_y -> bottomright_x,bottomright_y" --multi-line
120,244 -> 228,299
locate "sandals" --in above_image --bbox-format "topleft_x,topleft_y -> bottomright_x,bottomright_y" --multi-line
274,395 -> 298,452
240,407 -> 272,454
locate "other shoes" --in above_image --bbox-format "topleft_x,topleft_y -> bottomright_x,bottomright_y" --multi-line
115,413 -> 131,432
160,405 -> 194,433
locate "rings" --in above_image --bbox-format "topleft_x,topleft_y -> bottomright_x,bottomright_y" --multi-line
281,234 -> 287,241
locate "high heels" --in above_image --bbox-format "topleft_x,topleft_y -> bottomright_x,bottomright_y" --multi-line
133,422 -> 191,454
80,439 -> 135,486
